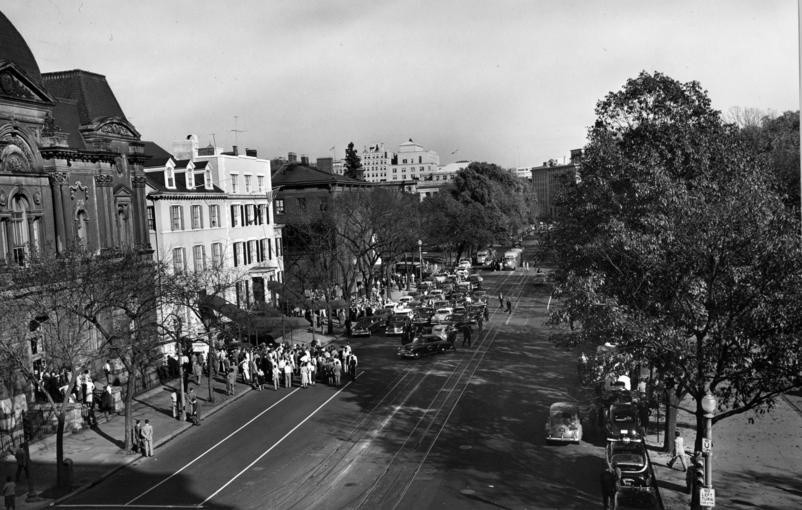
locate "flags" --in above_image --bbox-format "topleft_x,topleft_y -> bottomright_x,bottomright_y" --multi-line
266,188 -> 280,203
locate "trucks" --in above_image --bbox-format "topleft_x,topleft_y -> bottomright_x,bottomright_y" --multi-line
504,248 -> 523,270
477,249 -> 497,266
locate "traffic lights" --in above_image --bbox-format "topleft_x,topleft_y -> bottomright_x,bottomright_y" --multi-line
694,458 -> 705,487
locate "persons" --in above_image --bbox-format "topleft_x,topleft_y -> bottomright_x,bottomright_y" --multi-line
165,339 -> 358,424
15,444 -> 25,481
610,461 -> 621,508
36,359 -> 112,424
290,272 -> 415,342
600,464 -> 616,510
2,476 -> 17,510
141,419 -> 153,456
134,420 -> 142,453
448,292 -> 512,351
667,432 -> 687,472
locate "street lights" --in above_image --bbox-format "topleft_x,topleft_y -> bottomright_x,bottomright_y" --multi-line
417,240 -> 424,282
171,306 -> 189,421
695,390 -> 716,507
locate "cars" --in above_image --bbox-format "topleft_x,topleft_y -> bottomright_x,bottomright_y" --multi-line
350,254 -> 491,358
544,400 -> 584,442
595,341 -> 661,510
